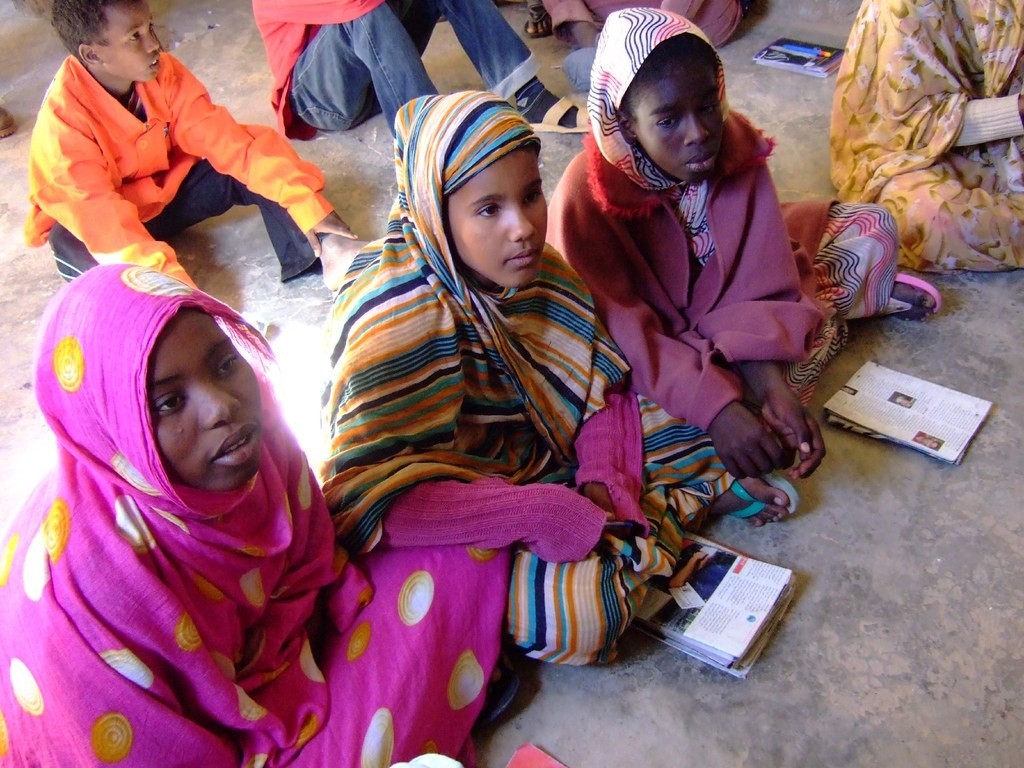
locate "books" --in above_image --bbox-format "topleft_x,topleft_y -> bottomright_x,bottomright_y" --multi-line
750,36 -> 844,78
820,360 -> 993,467
625,523 -> 798,681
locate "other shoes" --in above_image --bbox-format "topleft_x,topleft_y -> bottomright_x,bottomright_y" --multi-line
475,665 -> 520,728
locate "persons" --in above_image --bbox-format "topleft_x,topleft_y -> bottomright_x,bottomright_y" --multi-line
23,0 -> 377,296
829,0 -> 1024,274
314,89 -> 805,666
251,0 -> 753,141
0,261 -> 522,768
543,7 -> 941,479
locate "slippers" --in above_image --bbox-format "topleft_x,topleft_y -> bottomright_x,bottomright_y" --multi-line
729,473 -> 799,518
523,3 -> 553,38
528,96 -> 593,133
880,273 -> 940,315
